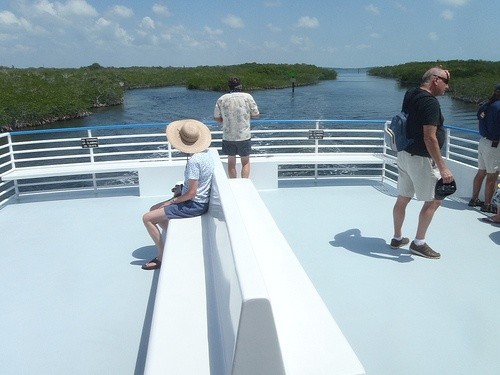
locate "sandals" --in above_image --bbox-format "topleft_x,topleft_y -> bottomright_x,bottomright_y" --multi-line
142,257 -> 162,270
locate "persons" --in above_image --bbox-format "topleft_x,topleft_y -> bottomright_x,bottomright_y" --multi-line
486,188 -> 500,223
142,118 -> 214,270
469,84 -> 500,214
390,66 -> 454,259
214,77 -> 259,178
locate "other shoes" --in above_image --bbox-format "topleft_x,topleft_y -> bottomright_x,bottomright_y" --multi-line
486,216 -> 500,223
468,198 -> 486,207
481,203 -> 497,214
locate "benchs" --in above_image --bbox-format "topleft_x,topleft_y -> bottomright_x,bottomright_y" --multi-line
142,148 -> 368,375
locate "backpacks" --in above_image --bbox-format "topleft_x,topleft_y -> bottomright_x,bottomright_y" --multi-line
390,93 -> 443,152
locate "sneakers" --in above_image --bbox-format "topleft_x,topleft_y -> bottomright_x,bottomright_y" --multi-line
409,240 -> 440,258
390,238 -> 410,248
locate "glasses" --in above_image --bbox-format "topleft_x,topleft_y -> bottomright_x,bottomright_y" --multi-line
433,74 -> 449,85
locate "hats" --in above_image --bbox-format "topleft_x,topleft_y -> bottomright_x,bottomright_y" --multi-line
435,178 -> 457,200
166,119 -> 211,153
